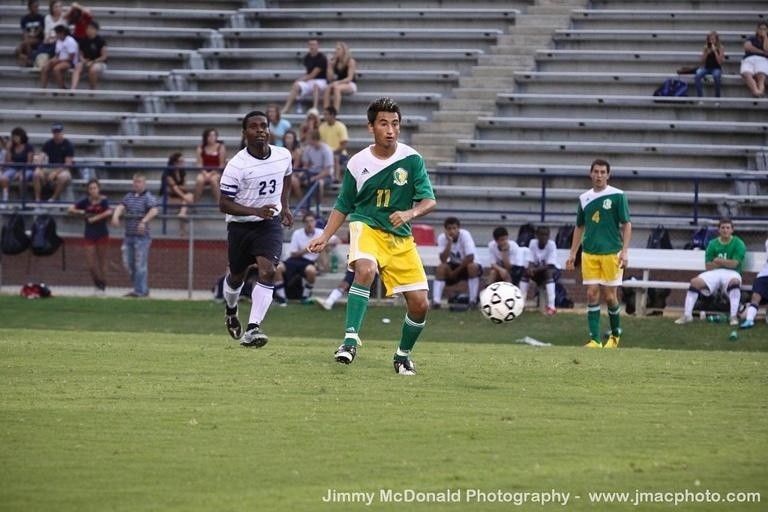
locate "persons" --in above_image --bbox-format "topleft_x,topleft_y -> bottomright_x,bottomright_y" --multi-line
1,2 -> 358,312
487,226 -> 524,287
739,21 -> 768,98
305,93 -> 437,377
738,238 -> 768,328
565,159 -> 631,349
430,216 -> 483,310
673,216 -> 746,326
518,225 -> 562,316
694,30 -> 725,97
218,110 -> 294,348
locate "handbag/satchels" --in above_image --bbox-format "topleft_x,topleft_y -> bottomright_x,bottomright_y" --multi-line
621,277 -> 671,317
447,291 -> 480,312
20,283 -> 51,300
694,291 -> 746,314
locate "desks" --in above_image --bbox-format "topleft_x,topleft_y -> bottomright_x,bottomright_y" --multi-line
584,247 -> 768,317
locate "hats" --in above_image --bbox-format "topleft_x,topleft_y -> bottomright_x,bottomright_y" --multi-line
51,121 -> 64,133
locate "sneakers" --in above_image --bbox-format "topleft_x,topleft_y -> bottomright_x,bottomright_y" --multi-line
738,318 -> 755,329
239,327 -> 268,349
674,314 -> 693,324
225,302 -> 243,340
333,342 -> 356,364
603,335 -> 620,349
584,339 -> 602,348
279,292 -> 332,310
393,354 -> 417,376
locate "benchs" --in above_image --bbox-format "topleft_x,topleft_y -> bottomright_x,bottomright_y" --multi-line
409,246 -> 578,299
280,243 -> 349,291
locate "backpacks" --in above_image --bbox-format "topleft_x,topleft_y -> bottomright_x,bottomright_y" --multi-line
652,79 -> 689,104
689,225 -> 712,249
28,216 -> 62,257
554,283 -> 574,309
647,225 -> 672,250
0,214 -> 30,255
517,221 -> 534,247
555,225 -> 575,248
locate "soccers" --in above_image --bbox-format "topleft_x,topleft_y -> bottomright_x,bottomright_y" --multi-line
739,303 -> 753,320
479,281 -> 524,324
